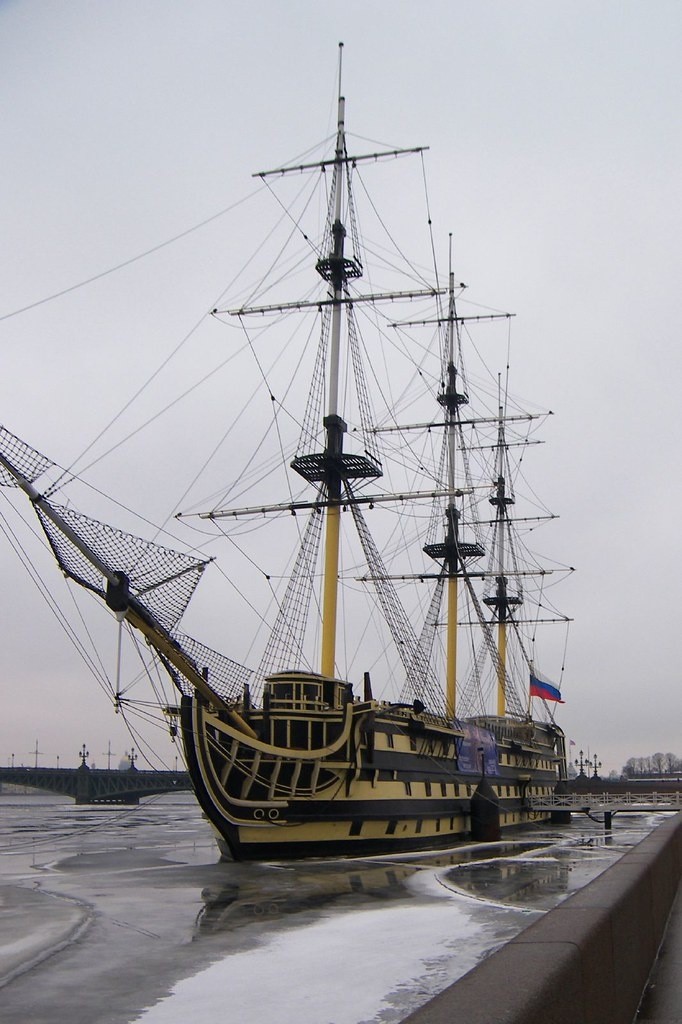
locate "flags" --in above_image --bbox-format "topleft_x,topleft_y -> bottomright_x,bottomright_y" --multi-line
528,663 -> 566,705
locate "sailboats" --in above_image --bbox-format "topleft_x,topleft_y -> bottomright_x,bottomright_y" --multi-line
0,42 -> 579,858
187,819 -> 577,943
